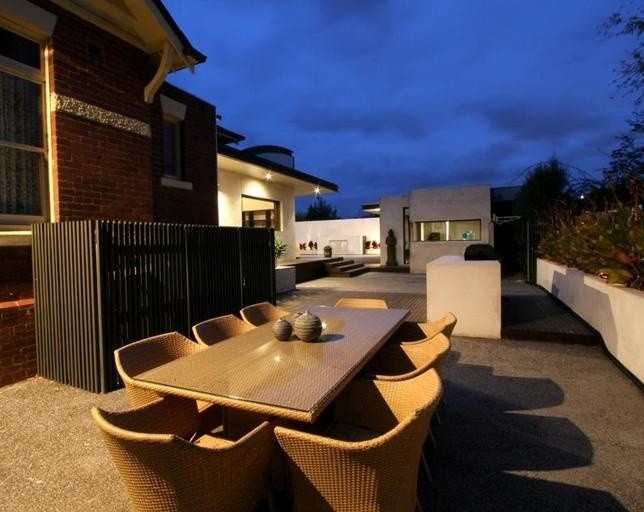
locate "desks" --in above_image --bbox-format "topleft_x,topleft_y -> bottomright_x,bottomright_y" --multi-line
131,306 -> 411,435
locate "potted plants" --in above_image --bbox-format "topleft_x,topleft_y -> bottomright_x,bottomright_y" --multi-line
324,245 -> 332,257
275,239 -> 289,268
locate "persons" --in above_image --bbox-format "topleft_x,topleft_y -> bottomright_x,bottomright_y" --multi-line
384,228 -> 397,266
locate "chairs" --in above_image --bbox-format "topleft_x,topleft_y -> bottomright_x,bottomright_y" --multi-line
333,298 -> 389,310
275,368 -> 442,510
115,331 -> 225,439
240,303 -> 290,328
387,312 -> 458,346
193,314 -> 252,349
358,333 -> 451,451
90,395 -> 286,511
